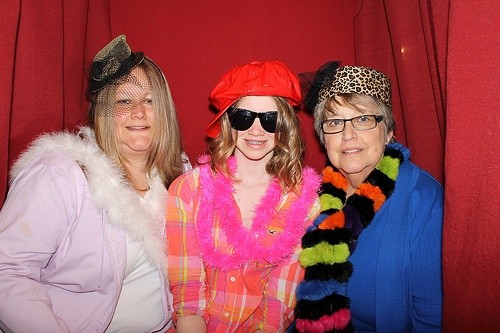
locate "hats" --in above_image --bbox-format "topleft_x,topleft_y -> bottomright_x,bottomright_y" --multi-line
297,60 -> 394,110
205,60 -> 302,140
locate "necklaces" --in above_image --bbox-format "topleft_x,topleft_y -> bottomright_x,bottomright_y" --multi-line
134,187 -> 149,192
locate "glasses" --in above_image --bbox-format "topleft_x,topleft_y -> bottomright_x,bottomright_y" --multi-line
224,106 -> 282,133
320,114 -> 384,134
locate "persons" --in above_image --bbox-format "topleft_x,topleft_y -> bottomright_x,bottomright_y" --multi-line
166,61 -> 321,333
0,33 -> 193,333
285,60 -> 443,333
